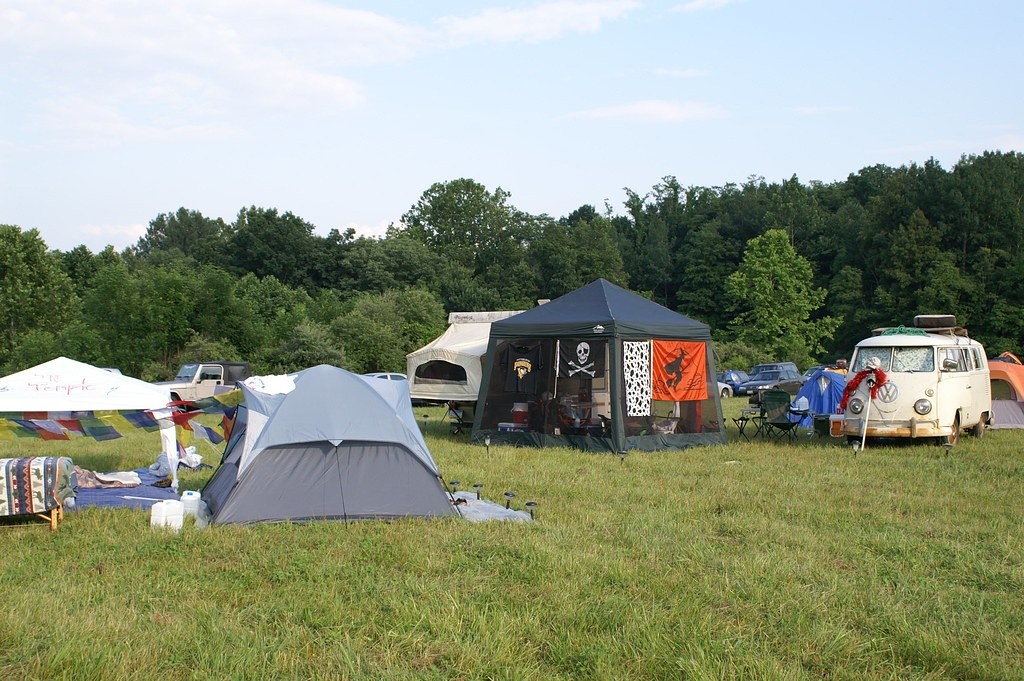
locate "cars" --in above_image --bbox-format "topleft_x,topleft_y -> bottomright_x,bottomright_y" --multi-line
801,366 -> 822,386
739,369 -> 808,397
363,373 -> 407,381
717,370 -> 750,399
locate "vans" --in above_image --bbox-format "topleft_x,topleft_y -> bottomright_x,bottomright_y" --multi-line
841,332 -> 995,447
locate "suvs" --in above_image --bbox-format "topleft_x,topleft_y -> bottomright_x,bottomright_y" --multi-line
748,361 -> 799,382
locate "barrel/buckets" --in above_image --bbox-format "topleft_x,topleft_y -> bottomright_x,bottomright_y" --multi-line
151,499 -> 183,530
511,403 -> 528,424
829,414 -> 844,437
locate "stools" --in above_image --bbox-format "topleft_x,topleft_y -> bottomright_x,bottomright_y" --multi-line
709,418 -> 726,430
752,414 -> 767,439
731,416 -> 750,442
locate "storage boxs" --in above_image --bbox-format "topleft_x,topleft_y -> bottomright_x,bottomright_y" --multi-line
497,422 -> 529,432
813,413 -> 845,438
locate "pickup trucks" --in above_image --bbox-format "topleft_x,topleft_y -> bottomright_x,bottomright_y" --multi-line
151,361 -> 252,403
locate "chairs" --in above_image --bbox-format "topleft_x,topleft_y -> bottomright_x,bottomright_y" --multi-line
556,406 -> 681,435
760,389 -> 809,444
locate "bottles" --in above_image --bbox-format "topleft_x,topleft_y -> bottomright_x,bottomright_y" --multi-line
575,418 -> 580,428
180,492 -> 200,517
837,404 -> 841,414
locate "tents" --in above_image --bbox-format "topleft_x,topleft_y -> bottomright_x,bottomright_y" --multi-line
470,278 -> 728,456
0,355 -> 185,495
196,364 -> 466,529
405,320 -> 530,401
988,352 -> 1024,429
785,369 -> 848,427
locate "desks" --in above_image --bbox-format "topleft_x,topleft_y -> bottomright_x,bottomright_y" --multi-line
0,455 -> 77,529
740,408 -> 762,437
560,401 -> 606,427
